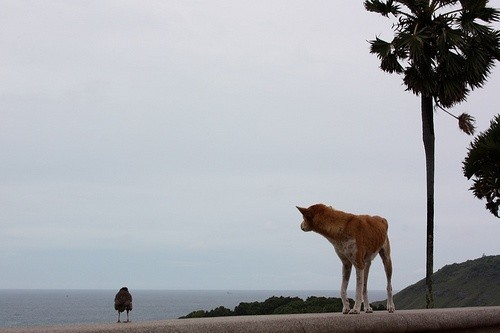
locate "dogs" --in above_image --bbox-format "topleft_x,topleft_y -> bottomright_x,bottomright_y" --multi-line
295,202 -> 395,314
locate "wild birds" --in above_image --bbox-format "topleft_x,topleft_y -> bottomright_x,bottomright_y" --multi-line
114,286 -> 133,323
